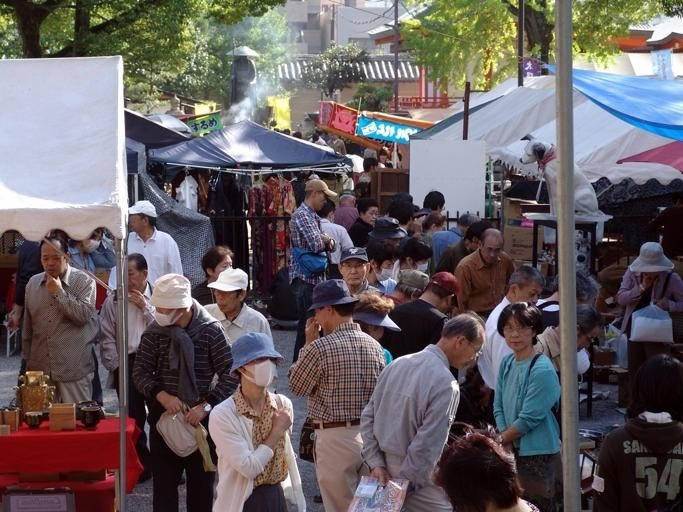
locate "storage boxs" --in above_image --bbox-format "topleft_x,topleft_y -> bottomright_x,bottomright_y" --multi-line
503,198 -> 543,260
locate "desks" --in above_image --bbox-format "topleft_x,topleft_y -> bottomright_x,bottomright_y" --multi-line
1,415 -> 145,512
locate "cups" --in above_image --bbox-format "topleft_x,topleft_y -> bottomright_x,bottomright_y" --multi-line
24,411 -> 44,429
0,407 -> 23,433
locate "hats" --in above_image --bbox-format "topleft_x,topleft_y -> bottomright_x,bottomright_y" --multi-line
398,269 -> 429,289
339,247 -> 369,266
629,243 -> 674,274
128,200 -> 156,218
352,311 -> 403,333
230,332 -> 286,381
306,280 -> 358,312
367,216 -> 408,239
429,272 -> 461,310
208,268 -> 248,291
150,274 -> 193,308
304,179 -> 338,197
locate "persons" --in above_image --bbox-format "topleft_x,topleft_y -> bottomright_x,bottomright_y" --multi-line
1,200 -> 308,512
283,131 -> 683,512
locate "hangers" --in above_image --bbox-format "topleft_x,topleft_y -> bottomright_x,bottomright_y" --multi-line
179,166 -> 316,185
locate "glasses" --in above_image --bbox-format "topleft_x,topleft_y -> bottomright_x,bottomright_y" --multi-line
457,336 -> 482,359
503,325 -> 533,332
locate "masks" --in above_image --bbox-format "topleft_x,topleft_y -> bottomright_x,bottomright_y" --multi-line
83,239 -> 100,253
239,359 -> 277,387
374,267 -> 393,282
152,308 -> 183,326
414,263 -> 428,273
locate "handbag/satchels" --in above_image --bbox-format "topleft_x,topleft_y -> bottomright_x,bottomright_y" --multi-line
156,402 -> 209,458
294,247 -> 329,277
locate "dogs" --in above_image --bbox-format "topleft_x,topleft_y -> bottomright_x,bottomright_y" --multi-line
518,133 -> 598,217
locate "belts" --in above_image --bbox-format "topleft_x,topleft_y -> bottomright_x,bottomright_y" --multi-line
311,419 -> 359,429
